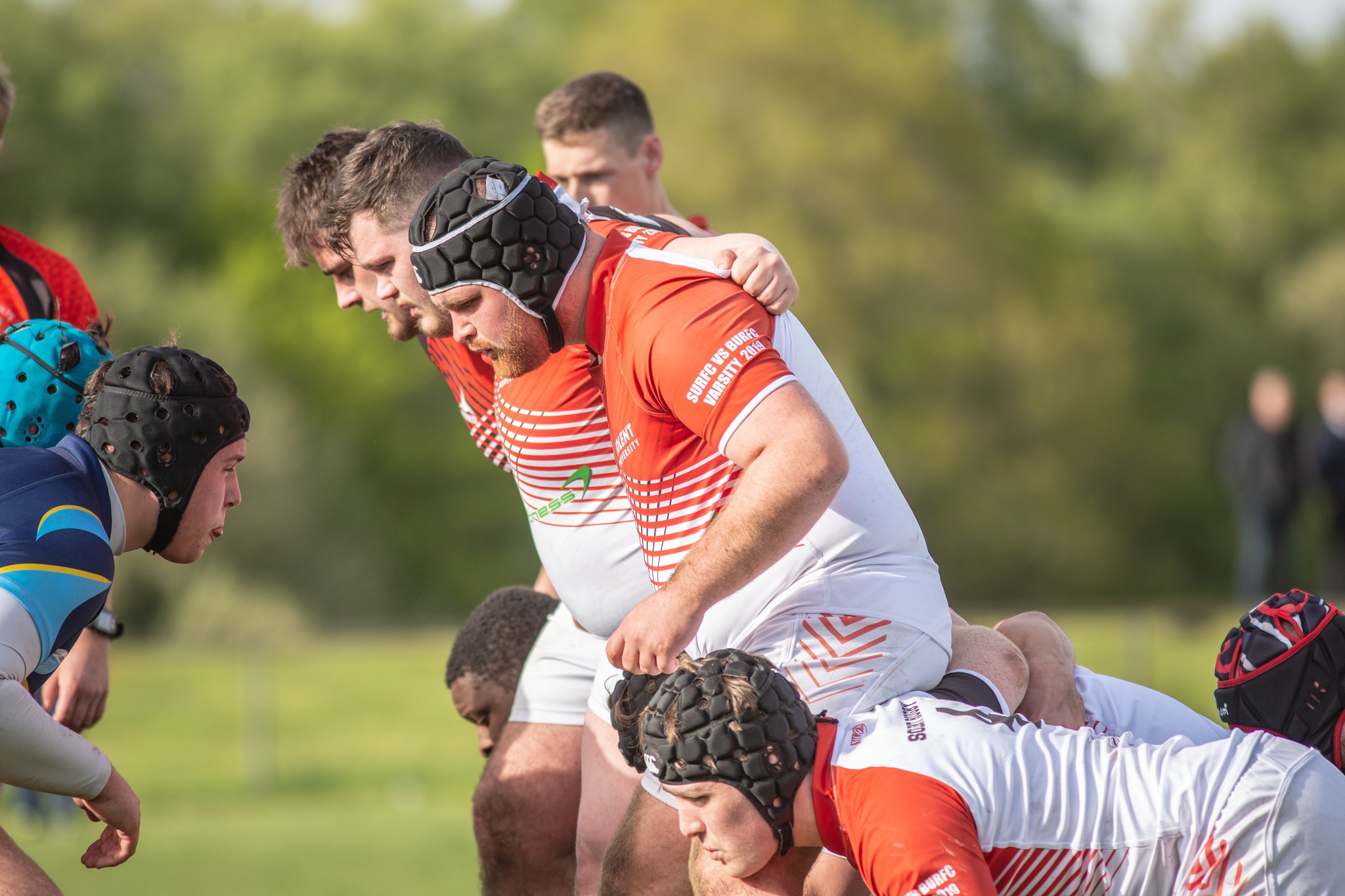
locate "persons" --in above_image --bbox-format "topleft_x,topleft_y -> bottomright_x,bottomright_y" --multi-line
0,73 -> 1345,896
410,156 -> 952,896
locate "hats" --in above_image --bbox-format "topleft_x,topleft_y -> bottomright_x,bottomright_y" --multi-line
0,319 -> 115,450
408,155 -> 586,319
1214,589 -> 1345,768
87,345 -> 252,511
640,647 -> 817,834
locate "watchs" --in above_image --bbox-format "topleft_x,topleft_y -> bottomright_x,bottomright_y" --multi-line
85,608 -> 124,638
